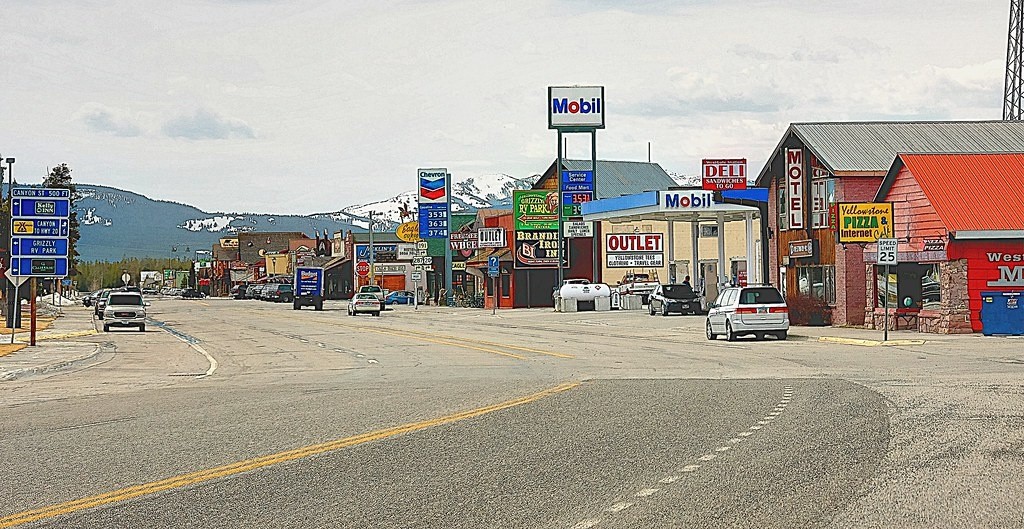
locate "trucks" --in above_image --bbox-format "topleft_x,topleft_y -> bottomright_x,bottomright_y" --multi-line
292,266 -> 325,310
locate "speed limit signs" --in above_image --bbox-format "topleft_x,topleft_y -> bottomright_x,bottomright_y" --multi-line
877,238 -> 899,266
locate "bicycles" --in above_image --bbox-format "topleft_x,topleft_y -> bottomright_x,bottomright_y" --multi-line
460,292 -> 485,308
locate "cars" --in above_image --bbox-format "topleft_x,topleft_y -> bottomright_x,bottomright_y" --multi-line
348,293 -> 380,316
386,290 -> 416,305
648,283 -> 701,316
121,286 -> 206,298
83,289 -> 125,319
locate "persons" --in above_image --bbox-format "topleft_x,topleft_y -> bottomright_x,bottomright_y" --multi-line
683,276 -> 690,286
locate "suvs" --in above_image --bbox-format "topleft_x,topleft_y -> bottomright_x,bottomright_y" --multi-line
230,285 -> 251,300
552,280 -> 591,303
100,292 -> 151,331
268,284 -> 293,303
246,284 -> 272,301
706,286 -> 789,340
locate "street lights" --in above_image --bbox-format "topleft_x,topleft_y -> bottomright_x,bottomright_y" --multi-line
272,257 -> 276,281
6,158 -> 15,187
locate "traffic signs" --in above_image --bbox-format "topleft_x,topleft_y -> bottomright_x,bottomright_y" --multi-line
10,188 -> 68,277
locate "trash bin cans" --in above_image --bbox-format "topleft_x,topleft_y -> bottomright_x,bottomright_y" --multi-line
980,292 -> 1024,336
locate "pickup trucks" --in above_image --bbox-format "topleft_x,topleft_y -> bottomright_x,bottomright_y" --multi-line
616,274 -> 660,302
356,285 -> 385,309
